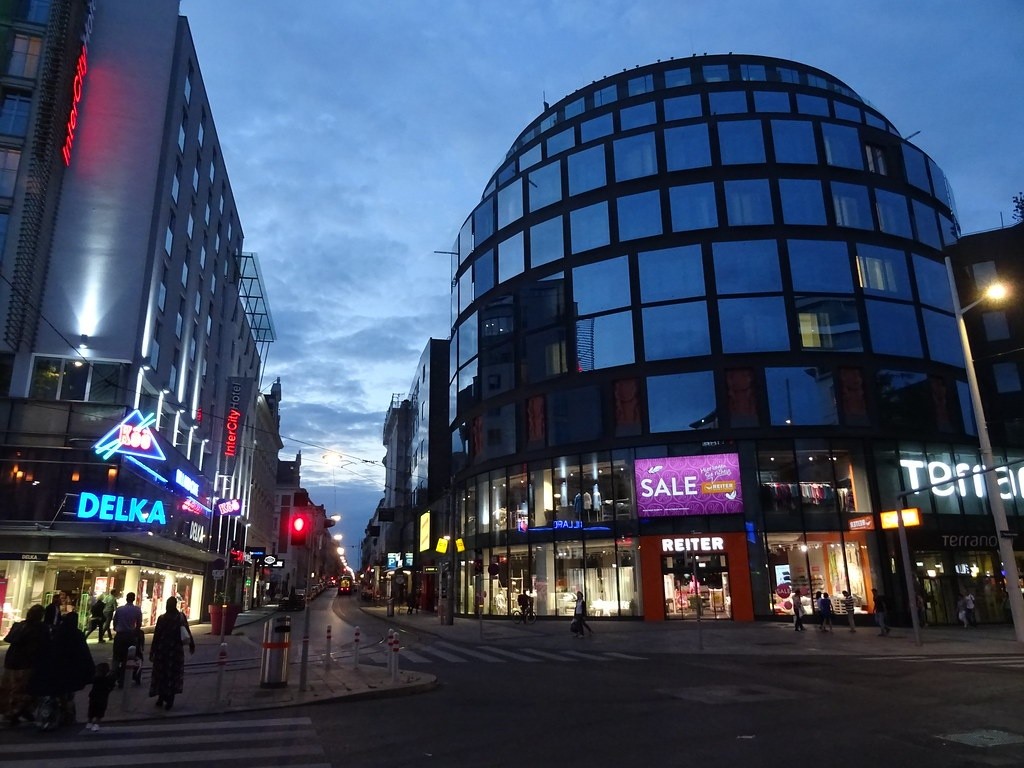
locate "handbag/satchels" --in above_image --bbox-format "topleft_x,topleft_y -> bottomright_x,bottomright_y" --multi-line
570,618 -> 579,632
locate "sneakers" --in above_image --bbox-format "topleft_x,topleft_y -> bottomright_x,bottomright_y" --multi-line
85,723 -> 92,729
92,723 -> 99,731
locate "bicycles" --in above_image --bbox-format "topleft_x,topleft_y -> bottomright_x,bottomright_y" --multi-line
513,607 -> 537,624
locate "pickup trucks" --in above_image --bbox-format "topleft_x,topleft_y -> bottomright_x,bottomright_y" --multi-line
338,580 -> 352,595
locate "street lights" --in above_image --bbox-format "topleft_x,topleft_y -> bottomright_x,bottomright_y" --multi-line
958,279 -> 1024,650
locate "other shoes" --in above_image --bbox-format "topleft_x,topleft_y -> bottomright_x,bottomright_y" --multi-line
165,703 -> 171,710
155,698 -> 163,706
794,622 -> 890,636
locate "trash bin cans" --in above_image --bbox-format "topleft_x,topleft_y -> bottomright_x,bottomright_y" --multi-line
386,598 -> 395,617
259,615 -> 291,689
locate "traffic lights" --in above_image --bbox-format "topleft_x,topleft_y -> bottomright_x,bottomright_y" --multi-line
290,492 -> 308,546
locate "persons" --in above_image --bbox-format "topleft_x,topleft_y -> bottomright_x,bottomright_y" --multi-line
821,593 -> 833,633
575,591 -> 593,638
816,591 -> 825,630
965,590 -> 976,628
957,594 -> 968,628
522,590 -> 528,624
593,489 -> 602,511
3,588 -> 144,732
583,492 -> 592,510
872,588 -> 890,636
1002,592 -> 1014,627
574,494 -> 582,514
793,589 -> 806,631
843,591 -> 856,632
917,593 -> 928,629
149,596 -> 195,710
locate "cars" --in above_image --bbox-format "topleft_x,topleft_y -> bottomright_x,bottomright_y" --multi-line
295,588 -> 306,602
